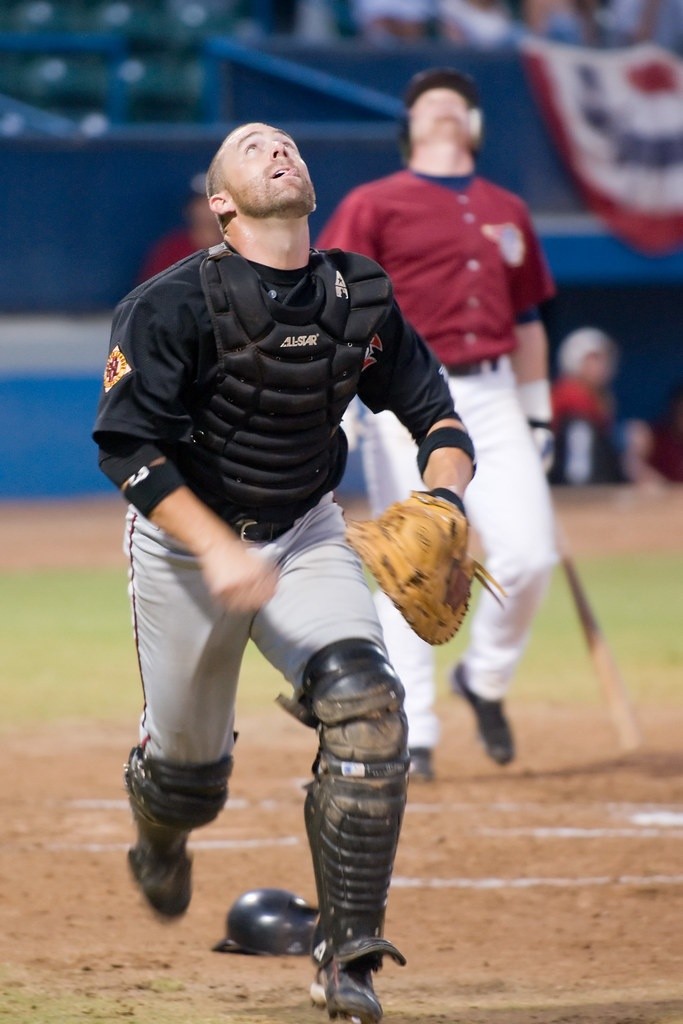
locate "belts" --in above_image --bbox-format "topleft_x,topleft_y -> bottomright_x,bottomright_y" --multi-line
445,360 -> 497,376
216,509 -> 293,543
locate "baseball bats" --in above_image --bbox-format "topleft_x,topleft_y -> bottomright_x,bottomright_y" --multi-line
555,533 -> 642,759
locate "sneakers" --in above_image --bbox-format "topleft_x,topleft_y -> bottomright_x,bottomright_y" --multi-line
322,959 -> 383,1024
450,665 -> 514,764
407,748 -> 432,781
128,821 -> 193,917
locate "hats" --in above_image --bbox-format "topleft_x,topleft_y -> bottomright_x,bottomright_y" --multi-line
403,67 -> 477,107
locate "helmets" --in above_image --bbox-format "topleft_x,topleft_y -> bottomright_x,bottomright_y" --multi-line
216,888 -> 322,955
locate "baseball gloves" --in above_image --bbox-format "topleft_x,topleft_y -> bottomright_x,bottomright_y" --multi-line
340,491 -> 506,650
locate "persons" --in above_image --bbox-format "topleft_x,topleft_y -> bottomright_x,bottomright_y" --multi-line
316,71 -> 560,783
138,0 -> 683,491
90,123 -> 476,1024
